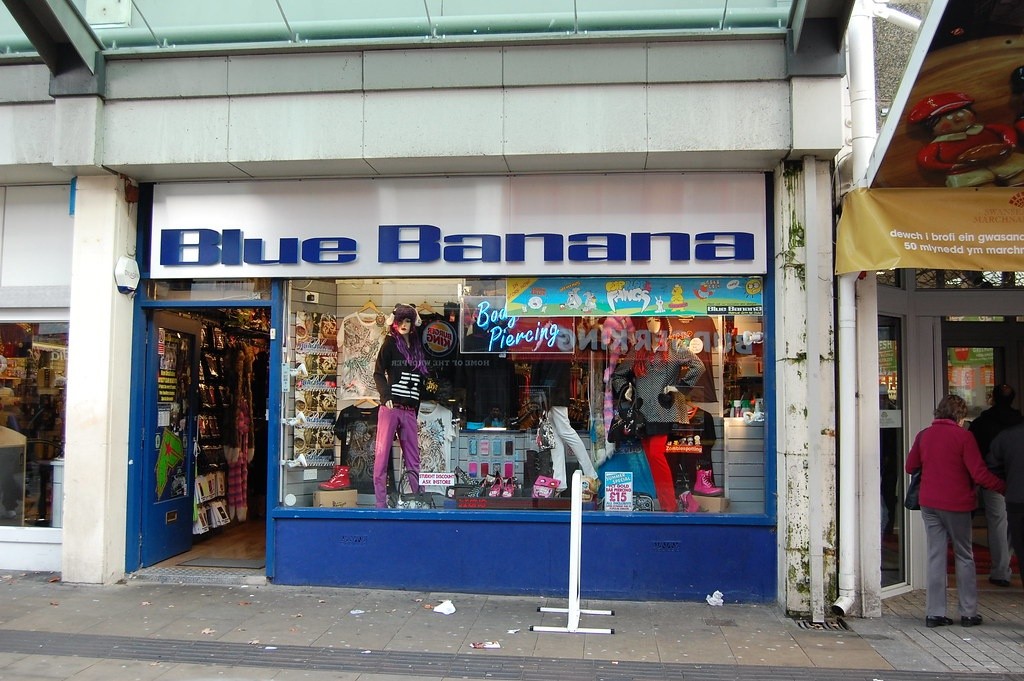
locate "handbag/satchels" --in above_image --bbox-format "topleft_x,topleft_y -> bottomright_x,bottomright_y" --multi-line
904,465 -> 922,509
386,470 -> 436,509
608,383 -> 648,442
536,410 -> 556,450
582,475 -> 598,503
531,476 -> 561,498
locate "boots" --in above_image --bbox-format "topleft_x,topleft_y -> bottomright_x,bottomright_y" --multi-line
679,491 -> 701,512
319,466 -> 350,490
694,470 -> 723,497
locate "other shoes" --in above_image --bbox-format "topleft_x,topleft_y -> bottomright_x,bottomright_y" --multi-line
961,614 -> 983,626
926,615 -> 953,627
454,466 -> 518,498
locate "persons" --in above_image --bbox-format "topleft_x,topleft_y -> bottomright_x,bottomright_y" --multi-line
7,395 -> 57,459
967,384 -> 1024,588
535,359 -> 601,498
611,316 -> 707,513
482,406 -> 507,429
903,395 -> 1003,628
374,305 -> 429,511
877,383 -> 899,536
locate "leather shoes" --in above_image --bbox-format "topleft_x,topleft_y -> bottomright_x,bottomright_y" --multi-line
989,577 -> 1009,586
